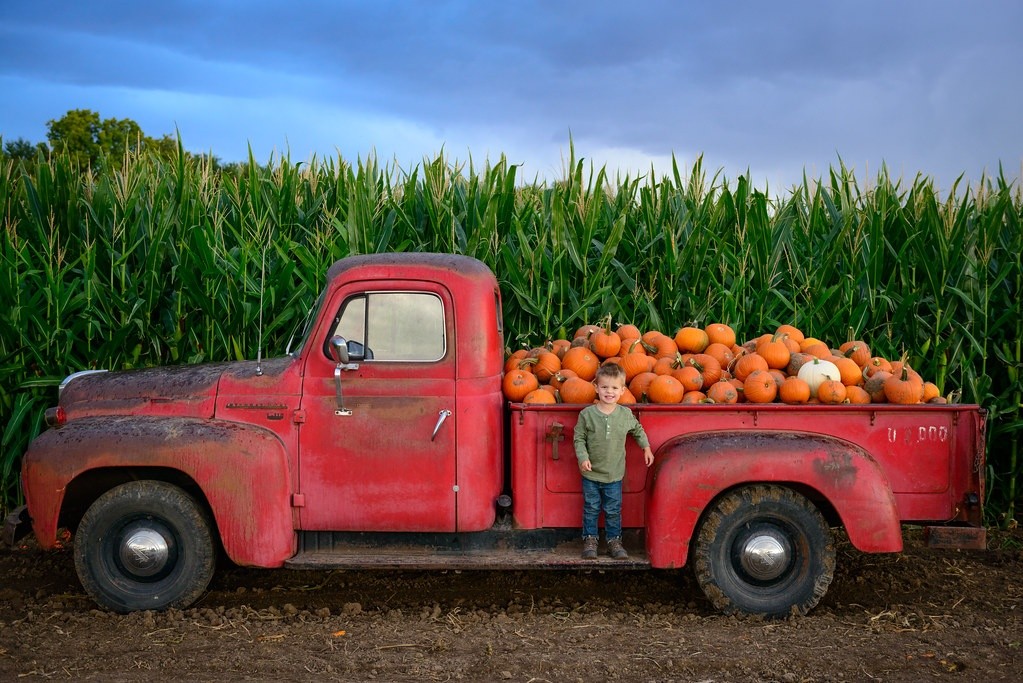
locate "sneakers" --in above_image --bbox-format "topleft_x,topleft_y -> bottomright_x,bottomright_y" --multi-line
582,536 -> 598,559
607,539 -> 629,559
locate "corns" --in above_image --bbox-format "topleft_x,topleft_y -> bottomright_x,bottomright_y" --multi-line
130,339 -> 142,356
937,349 -> 947,393
1003,341 -> 1017,384
175,317 -> 185,343
913,338 -> 930,370
557,326 -> 566,340
232,336 -> 244,361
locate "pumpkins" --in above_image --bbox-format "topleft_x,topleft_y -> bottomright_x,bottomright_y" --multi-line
504,310 -> 940,403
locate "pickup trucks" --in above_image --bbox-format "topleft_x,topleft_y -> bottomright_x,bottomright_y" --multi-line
16,249 -> 990,628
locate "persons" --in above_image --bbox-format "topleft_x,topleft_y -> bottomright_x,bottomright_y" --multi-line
574,363 -> 655,558
